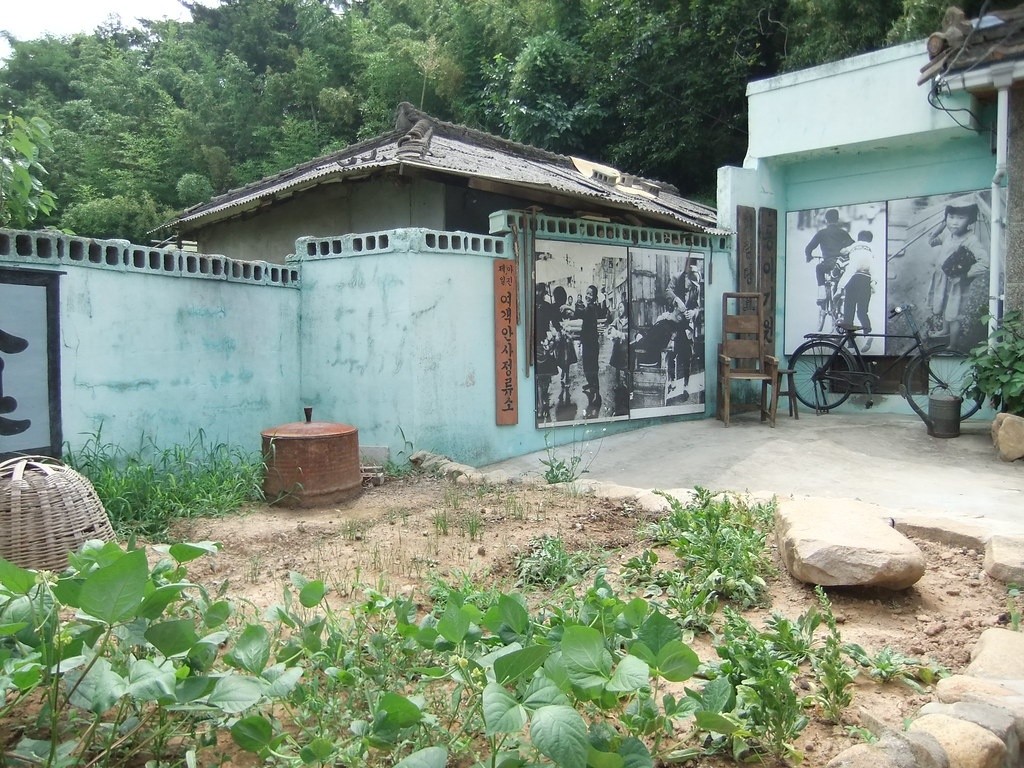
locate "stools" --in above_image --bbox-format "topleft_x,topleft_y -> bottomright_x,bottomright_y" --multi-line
768,370 -> 799,428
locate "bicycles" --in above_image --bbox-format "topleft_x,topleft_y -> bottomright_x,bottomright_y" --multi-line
787,303 -> 986,426
812,254 -> 848,333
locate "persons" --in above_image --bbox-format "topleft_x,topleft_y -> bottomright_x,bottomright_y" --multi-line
805,209 -> 878,353
535,265 -> 701,419
925,203 -> 990,357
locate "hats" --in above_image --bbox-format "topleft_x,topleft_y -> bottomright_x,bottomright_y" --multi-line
687,270 -> 699,287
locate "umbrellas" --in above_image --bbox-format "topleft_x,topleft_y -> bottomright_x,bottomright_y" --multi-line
534,258 -> 575,303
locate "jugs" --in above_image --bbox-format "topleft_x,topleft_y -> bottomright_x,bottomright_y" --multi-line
898,384 -> 964,438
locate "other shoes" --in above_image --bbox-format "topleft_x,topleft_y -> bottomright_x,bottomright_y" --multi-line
861,336 -> 873,353
817,298 -> 829,305
929,331 -> 950,338
560,372 -> 565,379
537,403 -> 549,416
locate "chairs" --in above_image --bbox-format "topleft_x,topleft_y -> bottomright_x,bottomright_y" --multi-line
716,292 -> 779,428
